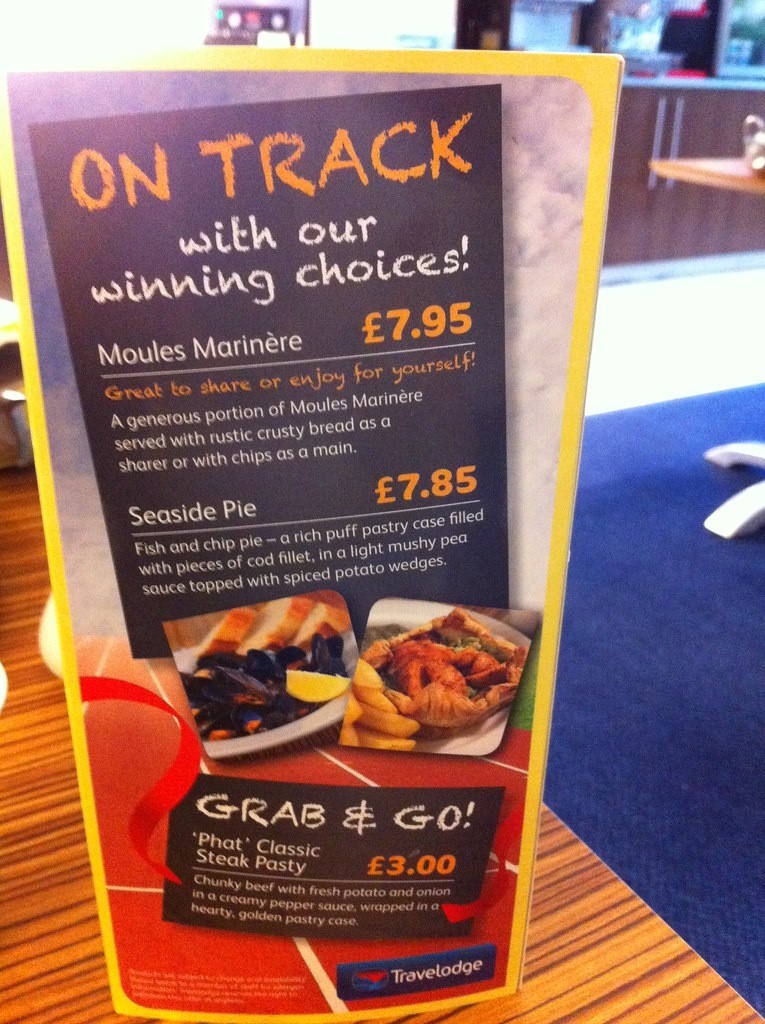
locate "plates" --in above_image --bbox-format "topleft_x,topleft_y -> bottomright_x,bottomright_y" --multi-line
369,600 -> 532,756
168,630 -> 353,757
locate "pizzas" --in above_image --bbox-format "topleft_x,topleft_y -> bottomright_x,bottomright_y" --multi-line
363,604 -> 529,741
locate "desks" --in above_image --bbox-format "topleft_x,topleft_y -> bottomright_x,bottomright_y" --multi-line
1,442 -> 764,1023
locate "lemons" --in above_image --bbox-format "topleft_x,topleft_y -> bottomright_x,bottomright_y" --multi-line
283,670 -> 352,702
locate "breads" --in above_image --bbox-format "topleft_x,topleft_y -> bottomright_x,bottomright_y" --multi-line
197,591 -> 351,656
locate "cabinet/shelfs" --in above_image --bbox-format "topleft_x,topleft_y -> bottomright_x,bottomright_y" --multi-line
598,78 -> 765,288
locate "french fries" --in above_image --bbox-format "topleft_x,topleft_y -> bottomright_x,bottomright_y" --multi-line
338,655 -> 420,751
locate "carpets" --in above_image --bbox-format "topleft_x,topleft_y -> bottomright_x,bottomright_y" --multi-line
539,377 -> 765,1023
